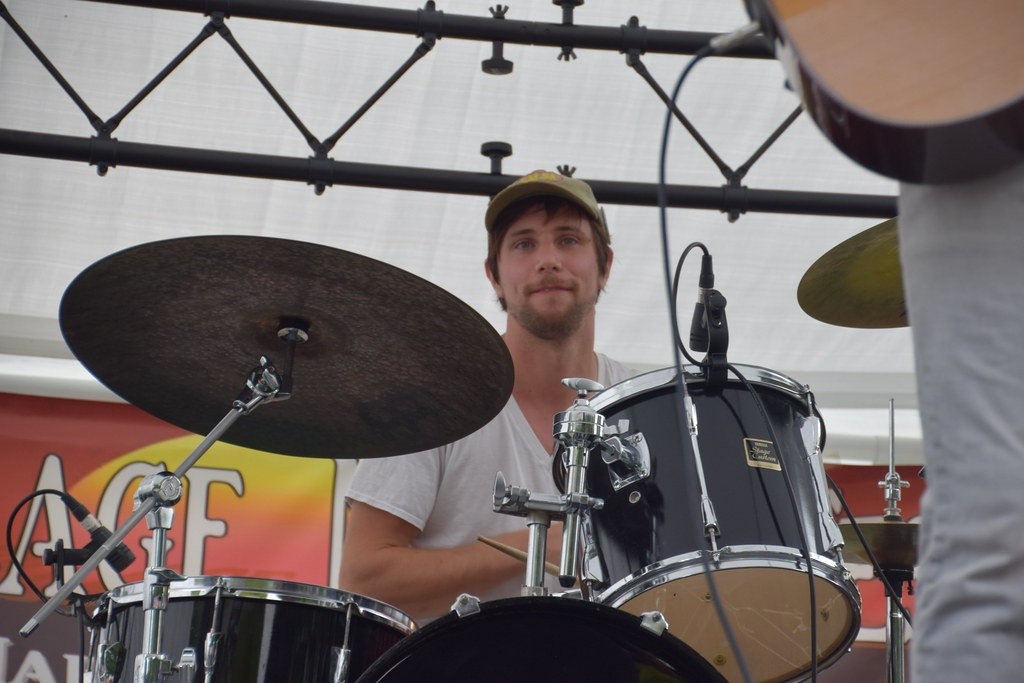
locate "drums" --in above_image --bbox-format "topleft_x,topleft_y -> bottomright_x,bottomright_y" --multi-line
91,575 -> 421,683
547,358 -> 864,683
356,593 -> 731,682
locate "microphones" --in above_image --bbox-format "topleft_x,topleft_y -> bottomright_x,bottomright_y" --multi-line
689,255 -> 714,353
61,492 -> 136,574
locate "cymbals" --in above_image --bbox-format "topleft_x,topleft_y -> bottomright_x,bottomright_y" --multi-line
796,213 -> 909,329
836,521 -> 923,567
58,232 -> 516,461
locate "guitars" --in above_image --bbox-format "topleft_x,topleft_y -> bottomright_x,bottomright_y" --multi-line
742,0 -> 1023,188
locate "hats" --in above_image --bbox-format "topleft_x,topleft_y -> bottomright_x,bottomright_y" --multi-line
485,170 -> 610,247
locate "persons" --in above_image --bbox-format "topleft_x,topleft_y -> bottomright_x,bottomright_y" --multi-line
894,172 -> 1024,683
337,168 -> 677,632
